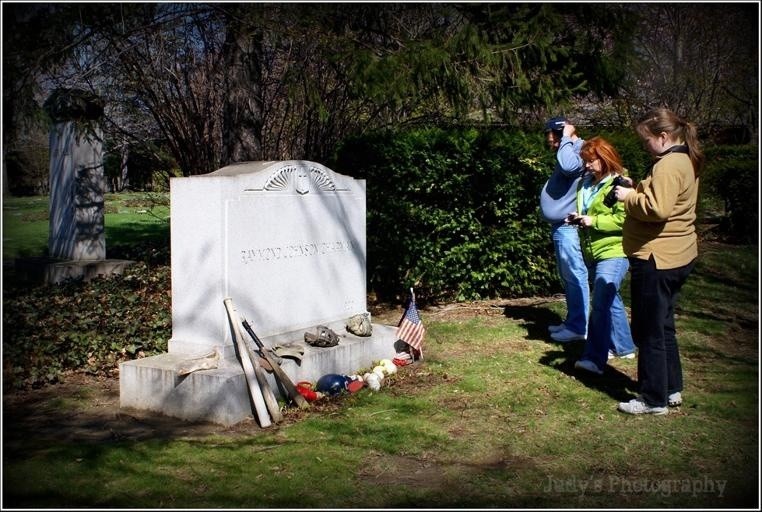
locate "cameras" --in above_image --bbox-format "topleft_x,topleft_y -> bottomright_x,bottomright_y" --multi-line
568,215 -> 583,226
603,174 -> 632,208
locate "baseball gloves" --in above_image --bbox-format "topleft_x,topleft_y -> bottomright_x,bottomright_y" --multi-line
346,313 -> 372,337
304,325 -> 339,347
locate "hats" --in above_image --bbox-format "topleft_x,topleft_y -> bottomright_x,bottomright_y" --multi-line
543,116 -> 572,131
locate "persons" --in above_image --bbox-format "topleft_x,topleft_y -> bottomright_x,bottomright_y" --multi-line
103,143 -> 152,194
224,120 -> 262,167
540,108 -> 703,415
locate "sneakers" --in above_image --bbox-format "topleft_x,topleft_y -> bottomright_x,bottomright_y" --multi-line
548,323 -> 588,342
618,392 -> 682,417
574,358 -> 604,374
608,347 -> 635,360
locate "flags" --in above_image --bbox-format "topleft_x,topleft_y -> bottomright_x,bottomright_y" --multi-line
396,300 -> 428,351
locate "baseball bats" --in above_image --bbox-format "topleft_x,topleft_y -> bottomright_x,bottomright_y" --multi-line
224,297 -> 310,428
